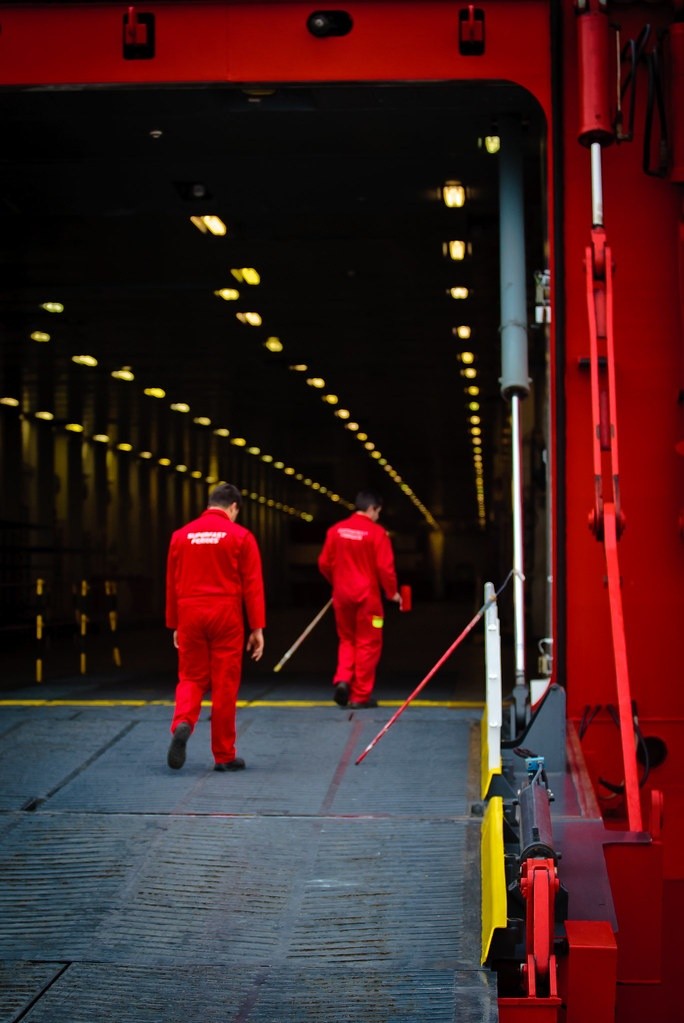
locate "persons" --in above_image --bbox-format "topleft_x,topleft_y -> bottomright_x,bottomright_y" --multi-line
318,489 -> 402,709
164,485 -> 266,770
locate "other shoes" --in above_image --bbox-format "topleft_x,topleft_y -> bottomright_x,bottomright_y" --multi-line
168,721 -> 190,769
215,756 -> 247,771
349,699 -> 378,708
334,682 -> 347,708
